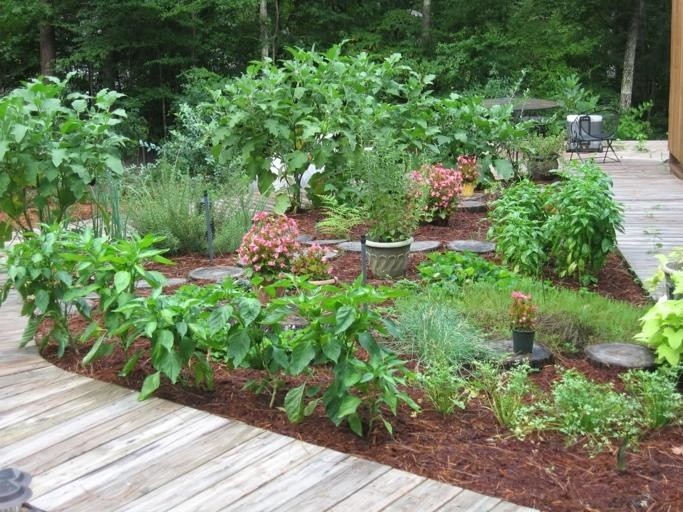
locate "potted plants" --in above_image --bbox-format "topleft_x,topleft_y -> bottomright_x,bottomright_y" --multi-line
361,150 -> 429,279
517,129 -> 566,180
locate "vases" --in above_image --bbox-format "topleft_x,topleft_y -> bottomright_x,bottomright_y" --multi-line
433,214 -> 450,226
465,175 -> 474,182
258,279 -> 337,306
513,333 -> 533,354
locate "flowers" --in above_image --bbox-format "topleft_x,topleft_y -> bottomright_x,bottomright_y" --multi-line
507,291 -> 538,332
404,162 -> 467,223
235,210 -> 340,280
455,153 -> 483,176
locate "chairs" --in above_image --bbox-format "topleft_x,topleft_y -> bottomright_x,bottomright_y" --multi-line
570,110 -> 621,163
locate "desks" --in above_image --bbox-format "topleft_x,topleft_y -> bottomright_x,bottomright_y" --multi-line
484,98 -> 559,138
